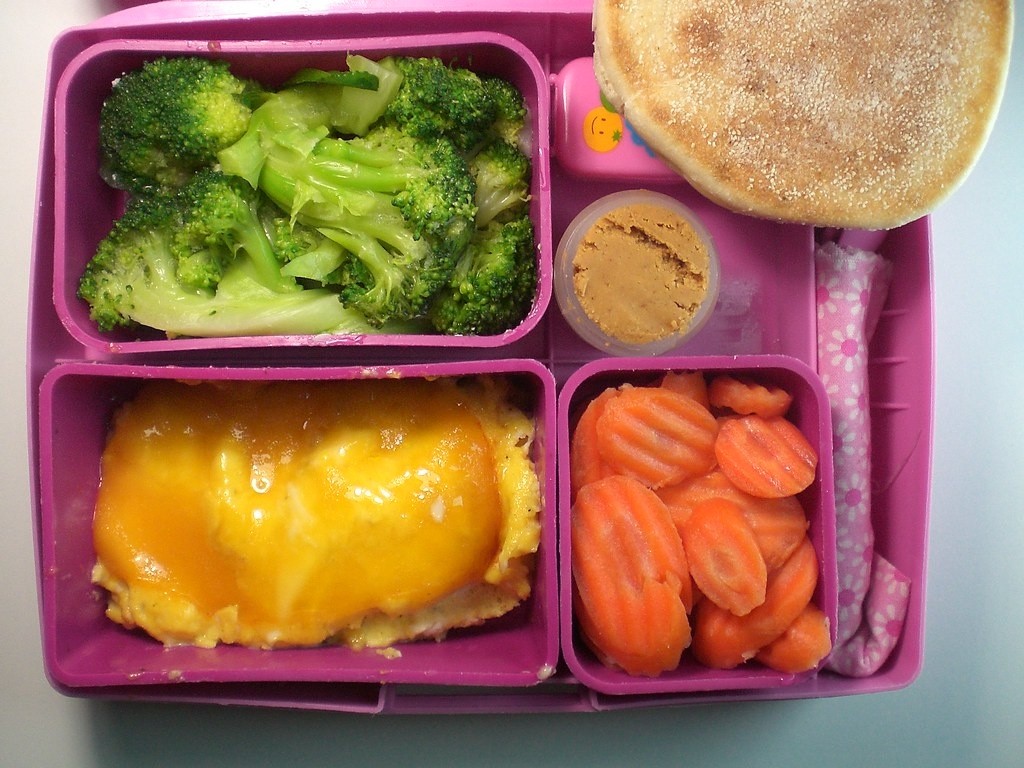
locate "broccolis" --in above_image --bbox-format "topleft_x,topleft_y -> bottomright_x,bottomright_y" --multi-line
80,50 -> 534,338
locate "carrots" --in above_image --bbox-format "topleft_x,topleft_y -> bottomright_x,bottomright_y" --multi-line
572,370 -> 831,677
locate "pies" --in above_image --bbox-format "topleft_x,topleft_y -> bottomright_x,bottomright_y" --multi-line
591,0 -> 1013,232
